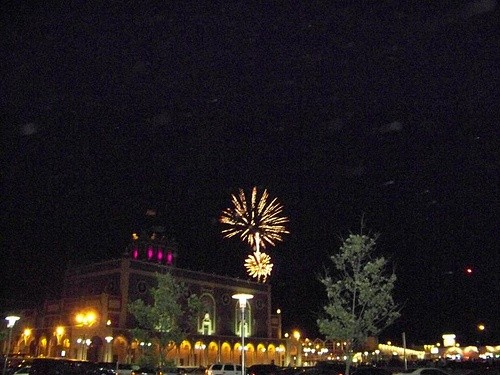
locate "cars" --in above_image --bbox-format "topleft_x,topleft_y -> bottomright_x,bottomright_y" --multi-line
3,358 -> 500,374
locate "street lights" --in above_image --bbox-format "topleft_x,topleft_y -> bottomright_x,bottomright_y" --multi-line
231,293 -> 255,375
